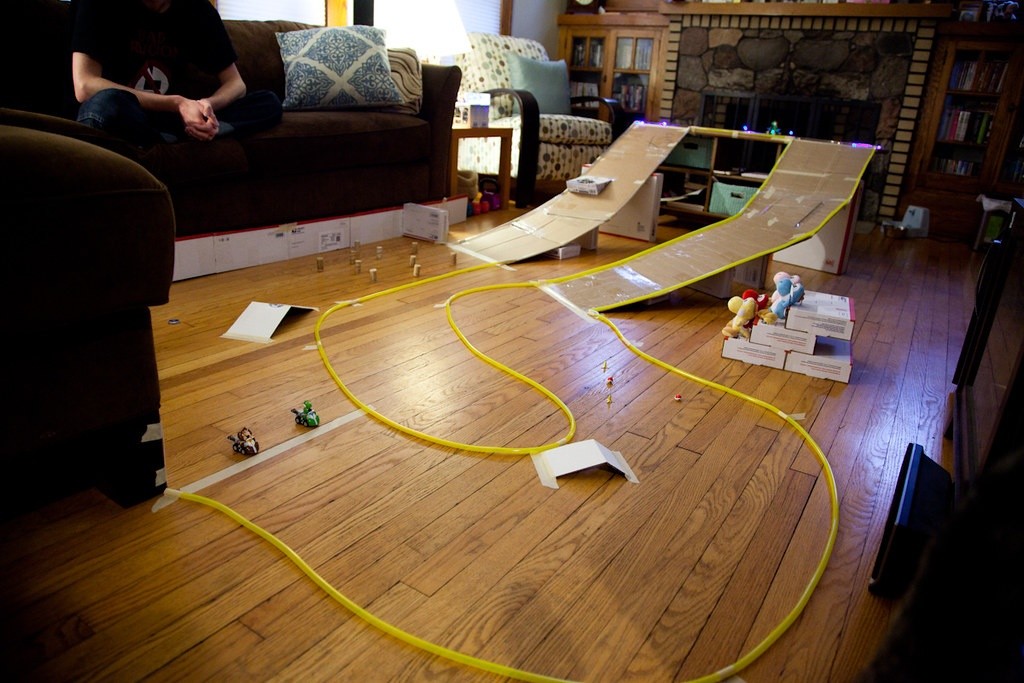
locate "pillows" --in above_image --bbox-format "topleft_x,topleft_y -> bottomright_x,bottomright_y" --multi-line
505,49 -> 574,116
274,25 -> 407,111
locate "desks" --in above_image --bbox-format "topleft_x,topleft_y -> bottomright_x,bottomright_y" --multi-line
447,123 -> 514,210
637,121 -> 890,225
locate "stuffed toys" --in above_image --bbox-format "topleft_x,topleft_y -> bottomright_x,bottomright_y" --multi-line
722,272 -> 805,339
992,2 -> 1021,20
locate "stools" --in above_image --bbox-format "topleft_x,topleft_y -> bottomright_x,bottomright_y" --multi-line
972,197 -> 1013,253
901,205 -> 930,238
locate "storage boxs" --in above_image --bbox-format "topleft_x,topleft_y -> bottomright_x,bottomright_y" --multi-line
683,252 -> 771,300
582,164 -> 664,242
720,289 -> 857,384
706,182 -> 758,214
540,226 -> 599,261
663,135 -> 711,170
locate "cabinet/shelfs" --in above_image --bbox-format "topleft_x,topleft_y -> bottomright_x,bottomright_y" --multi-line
563,26 -> 660,141
895,19 -> 1024,242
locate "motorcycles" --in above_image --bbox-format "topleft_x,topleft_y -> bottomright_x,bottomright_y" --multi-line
227,434 -> 259,455
291,408 -> 319,427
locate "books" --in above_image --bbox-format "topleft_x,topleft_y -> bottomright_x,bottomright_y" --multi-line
929,58 -> 1024,189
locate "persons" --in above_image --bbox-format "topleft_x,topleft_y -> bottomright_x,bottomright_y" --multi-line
64,0 -> 283,141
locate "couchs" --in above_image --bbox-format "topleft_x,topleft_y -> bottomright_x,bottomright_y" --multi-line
1,108 -> 177,523
451,30 -> 625,210
0,19 -> 464,237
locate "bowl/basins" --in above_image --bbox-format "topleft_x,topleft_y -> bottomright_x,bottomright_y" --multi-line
883,224 -> 908,238
